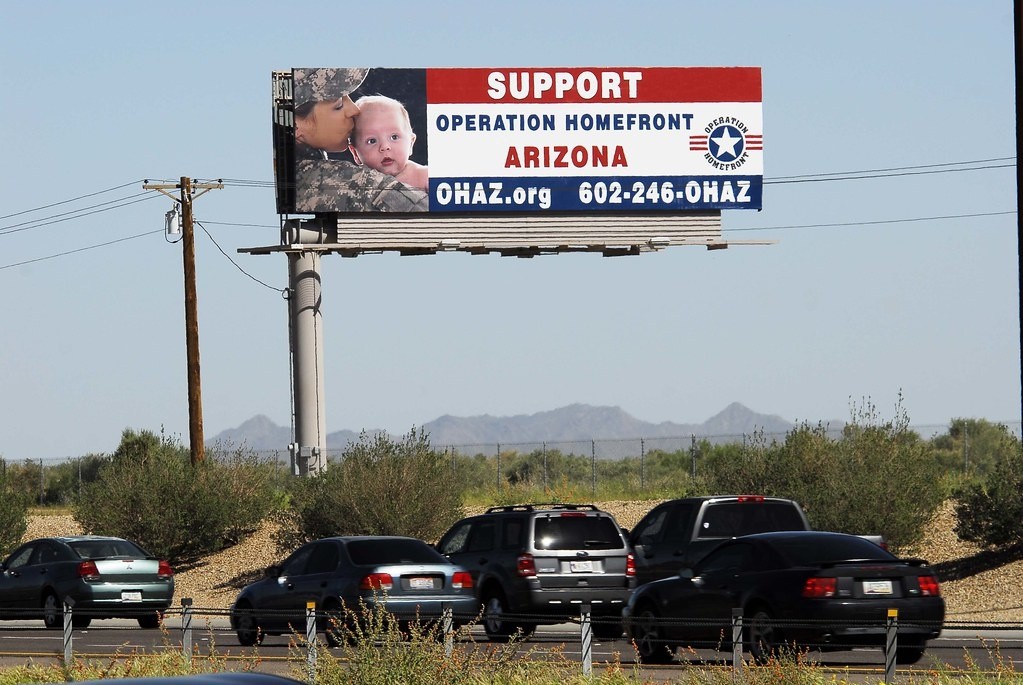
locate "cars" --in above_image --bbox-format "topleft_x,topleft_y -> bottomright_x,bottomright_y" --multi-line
623,530 -> 945,665
230,535 -> 481,648
0,534 -> 174,629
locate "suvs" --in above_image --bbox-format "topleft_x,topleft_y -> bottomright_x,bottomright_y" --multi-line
427,503 -> 634,644
617,495 -> 812,588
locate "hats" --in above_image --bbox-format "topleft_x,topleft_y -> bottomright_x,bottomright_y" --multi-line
292,69 -> 371,108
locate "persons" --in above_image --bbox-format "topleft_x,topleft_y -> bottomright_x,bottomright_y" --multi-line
292,67 -> 429,212
345,94 -> 428,192
